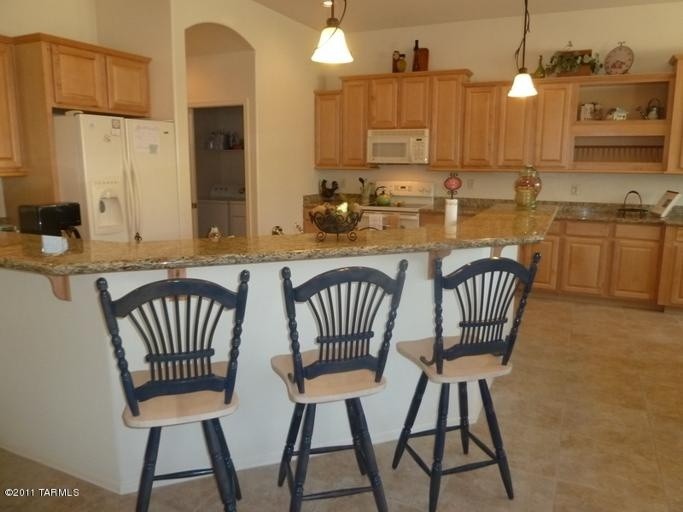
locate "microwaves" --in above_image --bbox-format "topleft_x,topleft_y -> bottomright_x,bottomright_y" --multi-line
366,128 -> 429,164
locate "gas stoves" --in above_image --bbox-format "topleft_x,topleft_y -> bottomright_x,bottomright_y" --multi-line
354,178 -> 436,213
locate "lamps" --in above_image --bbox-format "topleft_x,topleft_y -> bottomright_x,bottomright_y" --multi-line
309,2 -> 355,66
508,1 -> 538,99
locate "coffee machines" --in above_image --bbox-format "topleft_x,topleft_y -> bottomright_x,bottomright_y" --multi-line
19,199 -> 82,240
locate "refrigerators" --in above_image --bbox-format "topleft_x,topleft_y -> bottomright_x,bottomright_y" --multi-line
54,110 -> 180,242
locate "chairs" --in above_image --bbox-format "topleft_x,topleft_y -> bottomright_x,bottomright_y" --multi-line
270,260 -> 410,512
392,250 -> 542,511
96,271 -> 250,512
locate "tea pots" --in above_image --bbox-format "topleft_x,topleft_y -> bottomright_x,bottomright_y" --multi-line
636,97 -> 663,120
374,186 -> 393,206
605,107 -> 628,122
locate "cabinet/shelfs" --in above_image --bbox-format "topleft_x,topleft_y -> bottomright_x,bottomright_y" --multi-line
229,202 -> 247,239
529,238 -> 559,291
500,80 -> 535,167
47,41 -> 150,117
657,224 -> 683,309
536,81 -> 576,166
339,76 -> 368,169
427,70 -> 466,166
368,72 -> 433,129
560,218 -> 609,297
610,221 -> 657,305
0,36 -> 27,176
313,90 -> 342,170
466,83 -> 497,169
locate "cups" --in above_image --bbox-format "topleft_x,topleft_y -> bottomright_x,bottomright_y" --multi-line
41,234 -> 68,255
361,192 -> 369,203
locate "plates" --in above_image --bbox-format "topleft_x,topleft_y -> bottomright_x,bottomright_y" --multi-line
605,45 -> 634,75
